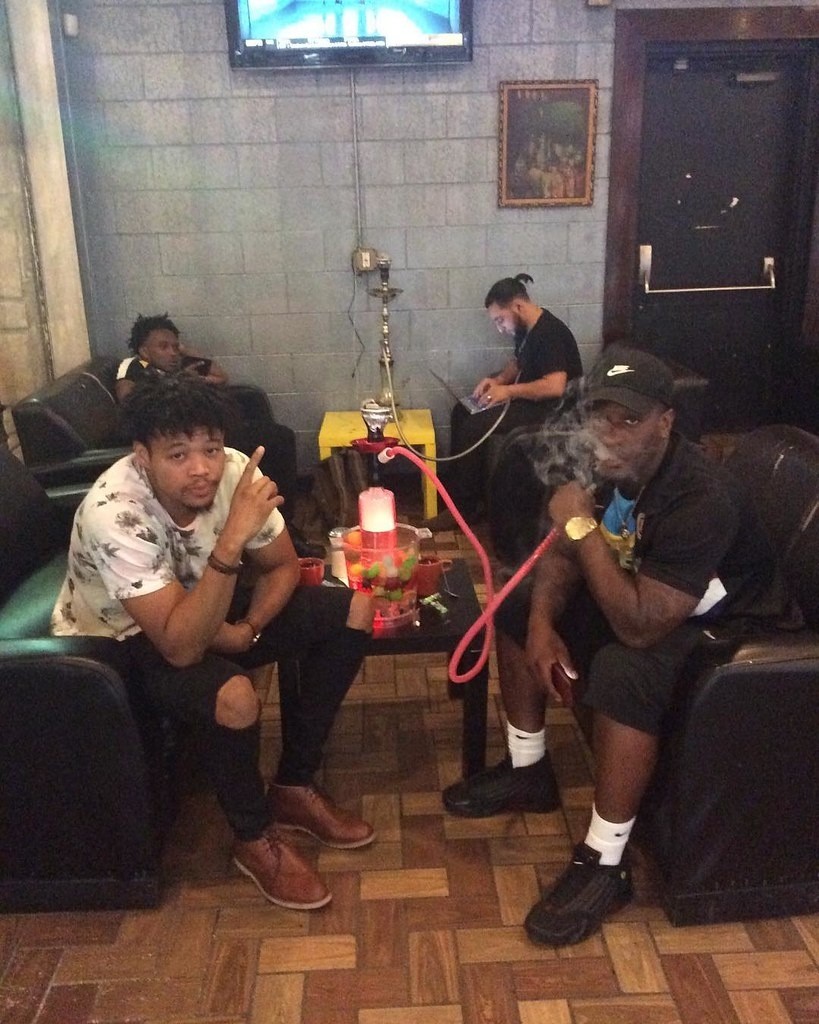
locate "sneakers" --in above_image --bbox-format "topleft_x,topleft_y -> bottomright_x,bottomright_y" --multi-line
443,748 -> 560,817
524,840 -> 633,946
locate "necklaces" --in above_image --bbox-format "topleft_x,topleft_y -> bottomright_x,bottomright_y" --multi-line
613,484 -> 646,540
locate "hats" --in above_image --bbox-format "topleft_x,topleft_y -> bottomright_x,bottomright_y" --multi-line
582,347 -> 675,412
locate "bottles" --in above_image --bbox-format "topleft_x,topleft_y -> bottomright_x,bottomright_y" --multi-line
328,527 -> 348,579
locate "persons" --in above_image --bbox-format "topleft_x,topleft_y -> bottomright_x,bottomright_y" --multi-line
115,314 -> 326,559
49,373 -> 377,909
417,274 -> 582,532
441,344 -> 807,948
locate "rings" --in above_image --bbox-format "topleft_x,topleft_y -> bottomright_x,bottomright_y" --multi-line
487,396 -> 491,399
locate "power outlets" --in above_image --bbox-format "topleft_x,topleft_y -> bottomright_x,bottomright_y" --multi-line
352,248 -> 378,273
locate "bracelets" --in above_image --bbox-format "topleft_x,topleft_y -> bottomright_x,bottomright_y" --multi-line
207,551 -> 244,575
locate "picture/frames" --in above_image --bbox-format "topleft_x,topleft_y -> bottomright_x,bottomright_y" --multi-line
500,78 -> 599,209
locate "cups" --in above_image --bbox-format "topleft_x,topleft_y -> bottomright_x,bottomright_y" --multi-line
418,556 -> 453,597
341,523 -> 432,628
298,557 -> 325,586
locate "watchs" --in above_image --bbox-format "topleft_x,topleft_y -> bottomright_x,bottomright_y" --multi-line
235,618 -> 262,654
564,516 -> 598,543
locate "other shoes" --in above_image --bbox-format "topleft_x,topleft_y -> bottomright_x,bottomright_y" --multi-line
438,496 -> 487,516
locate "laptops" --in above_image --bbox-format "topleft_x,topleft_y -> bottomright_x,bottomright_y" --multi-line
429,369 -> 508,414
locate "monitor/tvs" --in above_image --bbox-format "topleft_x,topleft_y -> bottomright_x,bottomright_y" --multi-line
224,0 -> 474,72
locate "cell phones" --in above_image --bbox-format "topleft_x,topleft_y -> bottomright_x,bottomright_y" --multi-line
552,665 -> 574,707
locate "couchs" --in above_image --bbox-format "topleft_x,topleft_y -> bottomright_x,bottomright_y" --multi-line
12,356 -> 274,488
483,335 -> 707,556
0,441 -> 202,915
570,423 -> 819,928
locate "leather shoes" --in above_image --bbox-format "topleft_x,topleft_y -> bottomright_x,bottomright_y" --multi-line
266,780 -> 377,848
235,832 -> 331,909
284,523 -> 328,557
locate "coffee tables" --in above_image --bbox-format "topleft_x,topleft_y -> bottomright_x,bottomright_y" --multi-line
277,557 -> 488,778
318,408 -> 437,520
